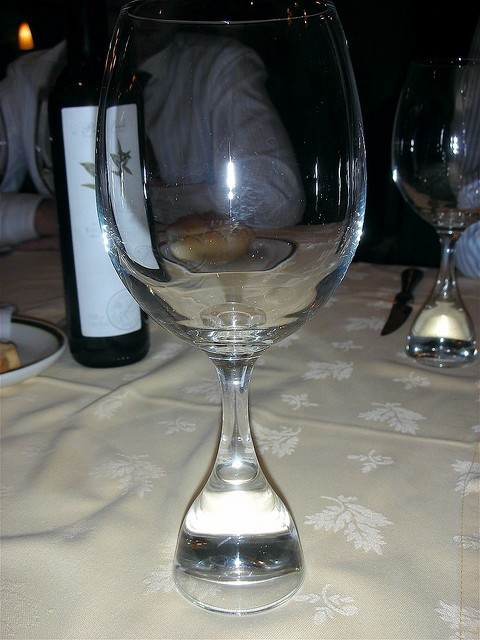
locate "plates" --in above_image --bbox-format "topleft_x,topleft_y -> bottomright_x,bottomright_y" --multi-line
152,233 -> 301,277
1,307 -> 69,391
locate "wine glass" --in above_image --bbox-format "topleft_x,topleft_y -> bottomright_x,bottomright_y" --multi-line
388,43 -> 480,372
91,0 -> 372,618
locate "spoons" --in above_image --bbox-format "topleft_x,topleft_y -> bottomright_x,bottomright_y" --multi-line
0,299 -> 20,344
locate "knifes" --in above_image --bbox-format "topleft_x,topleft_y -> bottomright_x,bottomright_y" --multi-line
379,265 -> 425,338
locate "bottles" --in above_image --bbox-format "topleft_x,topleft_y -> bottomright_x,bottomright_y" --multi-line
44,1 -> 152,370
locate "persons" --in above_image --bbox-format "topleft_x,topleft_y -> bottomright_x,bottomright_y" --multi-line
0,0 -> 305,246
